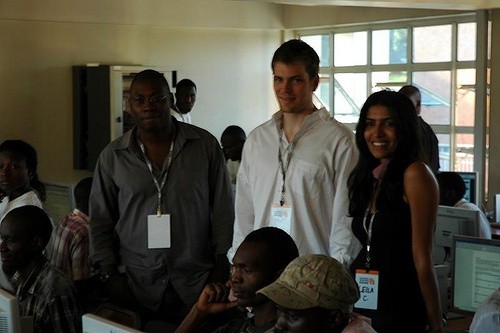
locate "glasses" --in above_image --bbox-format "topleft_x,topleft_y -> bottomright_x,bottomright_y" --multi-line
128,93 -> 171,105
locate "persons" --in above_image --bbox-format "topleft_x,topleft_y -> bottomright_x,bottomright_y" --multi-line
173,226 -> 300,333
398,85 -> 500,240
170,79 -> 197,124
226,40 -> 363,317
255,254 -> 377,333
0,139 -> 106,333
221,125 -> 246,191
347,90 -> 449,333
89,69 -> 235,333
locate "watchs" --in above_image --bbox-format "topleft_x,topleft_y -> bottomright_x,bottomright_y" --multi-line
97,271 -> 113,282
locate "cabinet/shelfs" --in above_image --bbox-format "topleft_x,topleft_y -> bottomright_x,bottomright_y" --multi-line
69,64 -> 186,171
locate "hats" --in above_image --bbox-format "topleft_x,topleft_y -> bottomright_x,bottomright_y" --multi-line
255,254 -> 361,313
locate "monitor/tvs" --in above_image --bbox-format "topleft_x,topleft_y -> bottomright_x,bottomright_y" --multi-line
41,182 -> 75,228
457,172 -> 479,206
450,234 -> 500,315
432,205 -> 479,267
82,313 -> 141,333
0,290 -> 21,333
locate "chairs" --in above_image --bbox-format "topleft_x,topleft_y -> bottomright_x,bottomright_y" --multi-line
37,179 -> 77,229
